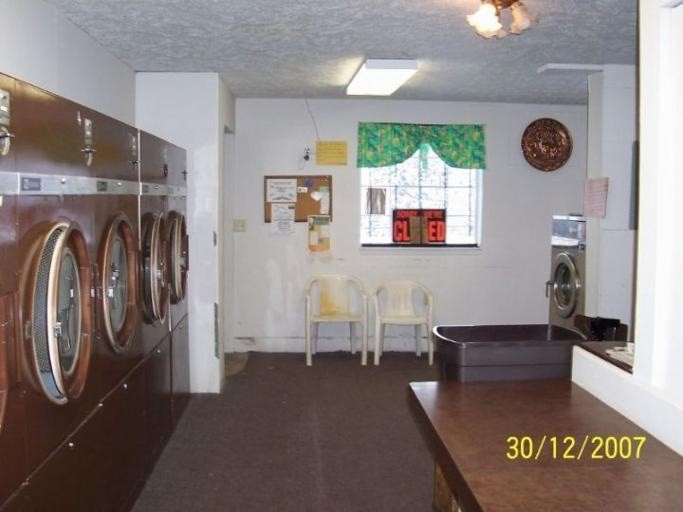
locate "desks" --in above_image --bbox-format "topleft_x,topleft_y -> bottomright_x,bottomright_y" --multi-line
406,377 -> 683,512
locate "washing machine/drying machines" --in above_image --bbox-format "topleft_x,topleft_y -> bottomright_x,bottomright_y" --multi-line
545,211 -> 585,328
0,70 -> 191,510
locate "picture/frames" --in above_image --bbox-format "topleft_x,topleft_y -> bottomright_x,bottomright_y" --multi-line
264,176 -> 332,222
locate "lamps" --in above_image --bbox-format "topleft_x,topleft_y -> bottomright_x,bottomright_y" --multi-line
465,1 -> 538,40
344,58 -> 419,96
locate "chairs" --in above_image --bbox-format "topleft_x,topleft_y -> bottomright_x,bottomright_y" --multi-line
301,273 -> 436,367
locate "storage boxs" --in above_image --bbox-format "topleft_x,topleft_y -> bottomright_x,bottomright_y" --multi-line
433,324 -> 588,383
